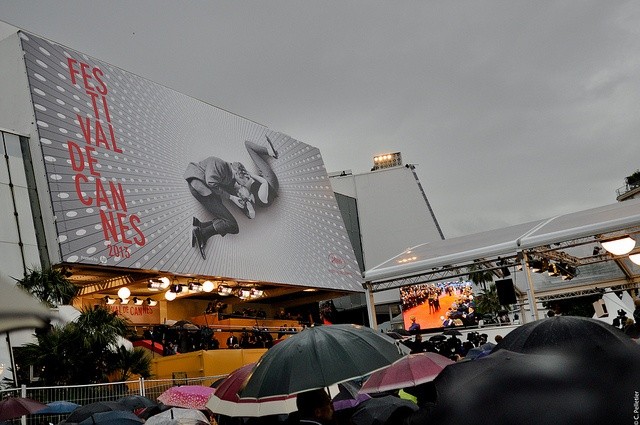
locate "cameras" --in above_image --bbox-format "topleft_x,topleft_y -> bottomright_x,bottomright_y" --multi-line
466,331 -> 488,345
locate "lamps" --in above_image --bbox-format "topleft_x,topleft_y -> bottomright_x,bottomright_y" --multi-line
105,276 -> 264,307
600,237 -> 636,255
629,252 -> 640,266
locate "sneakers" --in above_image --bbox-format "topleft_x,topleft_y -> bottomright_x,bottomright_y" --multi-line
265,136 -> 278,160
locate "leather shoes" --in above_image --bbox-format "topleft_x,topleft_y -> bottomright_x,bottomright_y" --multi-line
193,226 -> 206,260
192,217 -> 201,247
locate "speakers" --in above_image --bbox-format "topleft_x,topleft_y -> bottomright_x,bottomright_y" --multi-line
495,278 -> 517,305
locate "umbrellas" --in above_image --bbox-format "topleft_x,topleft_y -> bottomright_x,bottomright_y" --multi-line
35,400 -> 82,415
236,323 -> 411,401
143,407 -> 212,425
443,330 -> 463,335
157,385 -> 217,410
66,400 -> 133,422
490,316 -> 640,360
432,349 -> 557,405
357,351 -> 455,394
0,396 -> 49,421
206,361 -> 340,417
333,381 -> 372,411
80,411 -> 146,425
386,332 -> 403,339
115,395 -> 158,410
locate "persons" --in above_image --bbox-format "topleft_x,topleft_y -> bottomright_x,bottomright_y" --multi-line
296,388 -> 334,425
237,135 -> 279,221
285,312 -> 295,320
295,313 -> 302,322
226,332 -> 238,349
206,302 -> 216,313
274,311 -> 280,320
257,308 -> 266,318
413,329 -> 488,360
280,311 -> 285,319
495,335 -> 502,343
400,280 -> 475,330
183,157 -> 247,261
278,325 -> 285,339
624,318 -> 636,338
289,324 -> 297,332
283,324 -> 287,330
